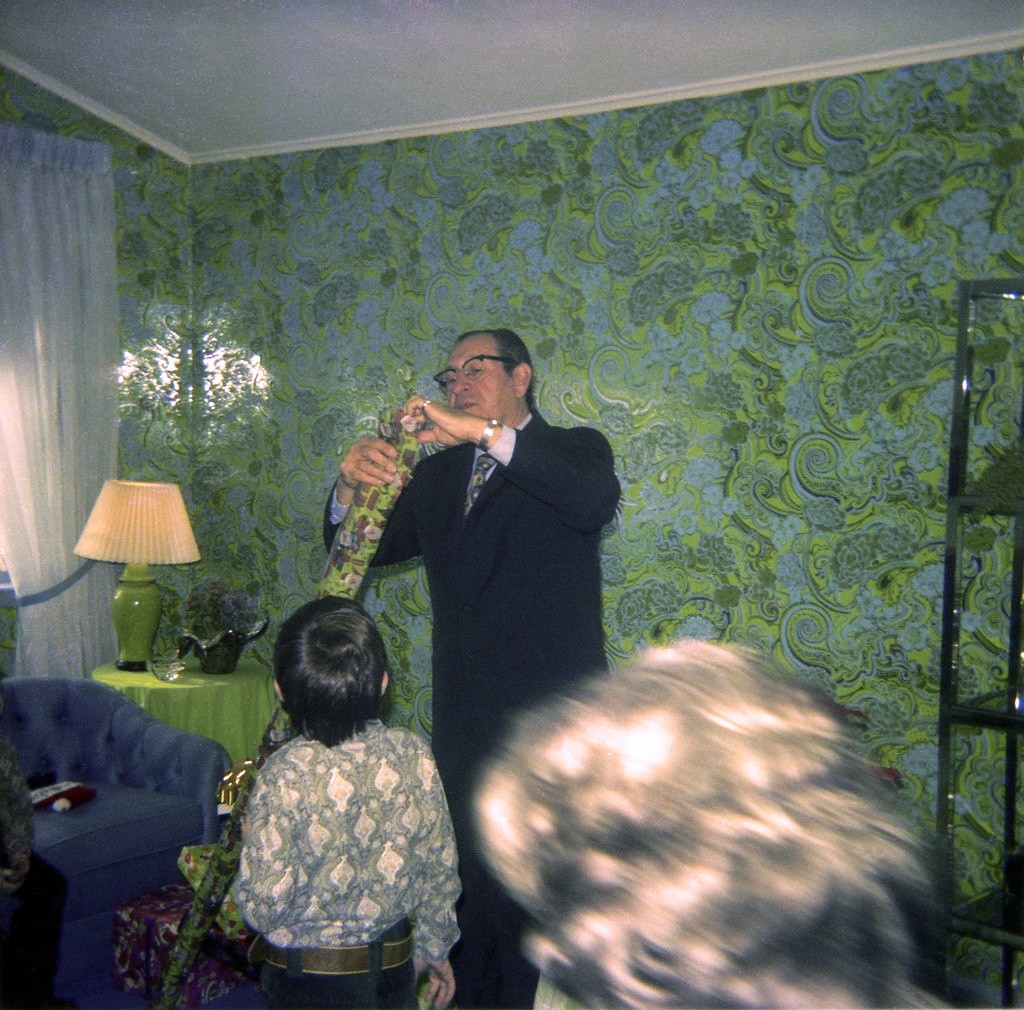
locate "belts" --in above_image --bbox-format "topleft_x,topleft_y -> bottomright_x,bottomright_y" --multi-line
245,934 -> 417,976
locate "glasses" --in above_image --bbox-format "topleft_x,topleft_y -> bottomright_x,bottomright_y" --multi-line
433,355 -> 518,388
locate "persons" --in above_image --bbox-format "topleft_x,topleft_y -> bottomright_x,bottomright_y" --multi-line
0,732 -> 78,1010
220,327 -> 971,1009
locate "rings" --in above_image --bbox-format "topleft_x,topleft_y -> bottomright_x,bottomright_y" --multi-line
421,400 -> 431,410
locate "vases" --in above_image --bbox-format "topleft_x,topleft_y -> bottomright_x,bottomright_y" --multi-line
184,628 -> 253,674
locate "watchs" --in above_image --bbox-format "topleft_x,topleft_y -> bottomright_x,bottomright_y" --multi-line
476,417 -> 503,452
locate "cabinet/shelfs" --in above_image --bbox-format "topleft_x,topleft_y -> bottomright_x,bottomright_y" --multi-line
930,281 -> 1024,1007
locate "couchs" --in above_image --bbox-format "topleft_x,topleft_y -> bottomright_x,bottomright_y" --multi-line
0,674 -> 233,1010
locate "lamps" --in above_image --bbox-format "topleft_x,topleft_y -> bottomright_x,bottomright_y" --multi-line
73,479 -> 200,672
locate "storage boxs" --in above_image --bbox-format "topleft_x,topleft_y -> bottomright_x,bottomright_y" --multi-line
108,841 -> 247,1007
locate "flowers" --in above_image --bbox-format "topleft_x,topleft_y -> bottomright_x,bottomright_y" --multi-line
182,580 -> 263,637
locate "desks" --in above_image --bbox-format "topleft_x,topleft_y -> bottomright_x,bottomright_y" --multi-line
91,667 -> 279,764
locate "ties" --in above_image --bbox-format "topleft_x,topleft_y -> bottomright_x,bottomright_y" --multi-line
464,453 -> 496,518
